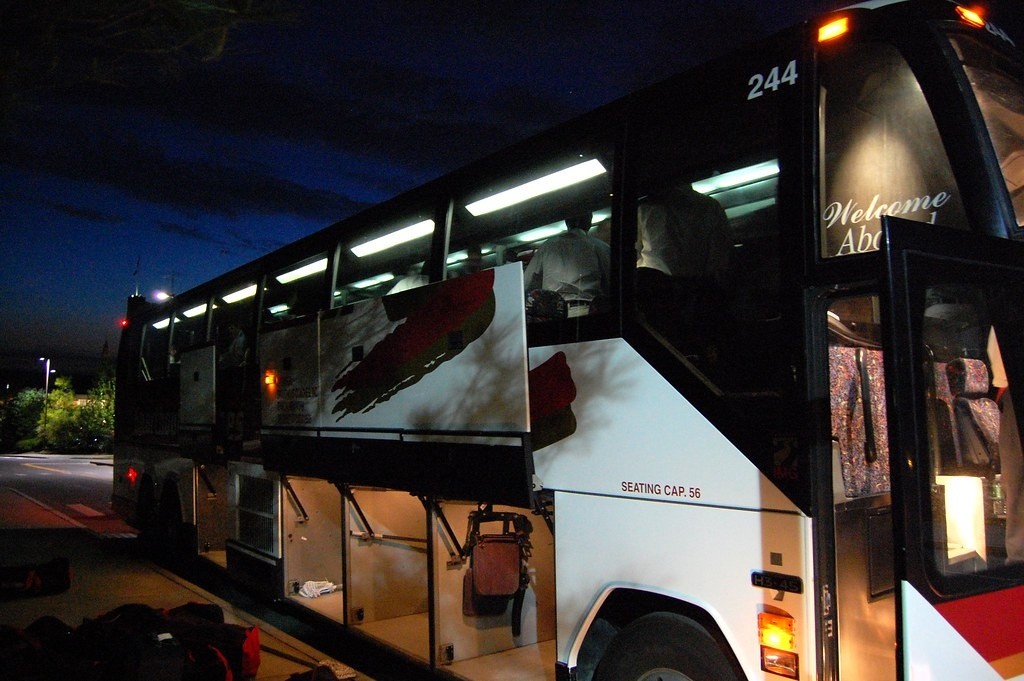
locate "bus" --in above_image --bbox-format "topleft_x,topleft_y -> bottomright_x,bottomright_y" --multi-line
112,1 -> 1024,681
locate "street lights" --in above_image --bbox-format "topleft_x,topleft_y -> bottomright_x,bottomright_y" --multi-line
39,357 -> 55,454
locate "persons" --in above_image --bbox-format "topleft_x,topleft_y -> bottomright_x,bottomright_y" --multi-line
221,323 -> 248,370
377,177 -> 739,320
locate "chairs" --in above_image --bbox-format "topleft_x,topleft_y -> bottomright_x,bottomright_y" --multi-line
945,358 -> 1003,475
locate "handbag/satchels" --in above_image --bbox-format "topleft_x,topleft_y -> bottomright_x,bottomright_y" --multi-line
0,599 -> 263,681
